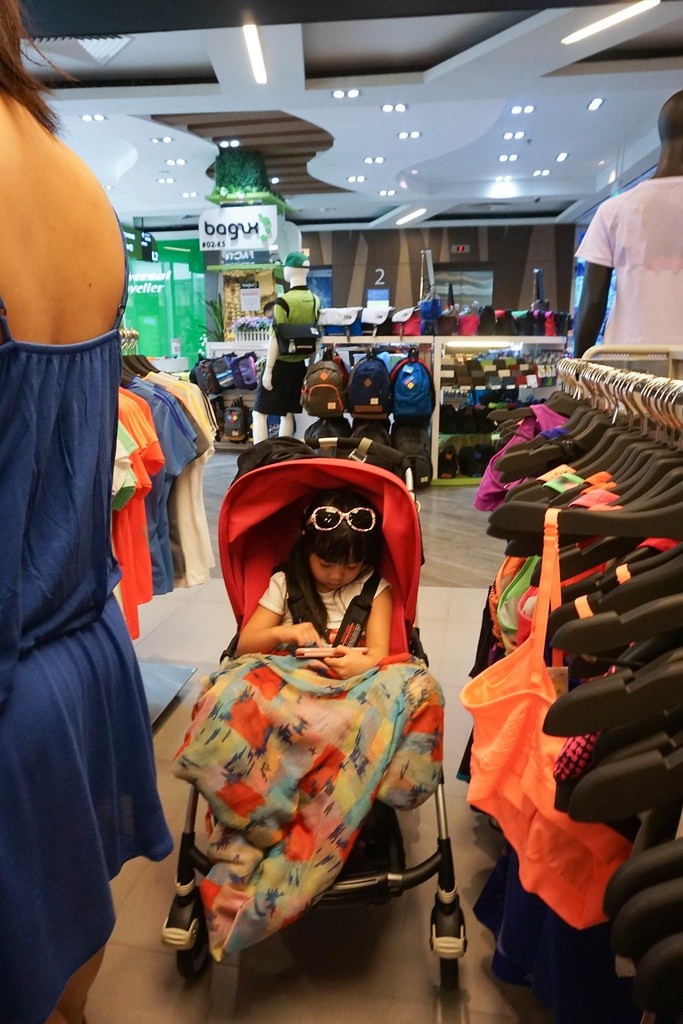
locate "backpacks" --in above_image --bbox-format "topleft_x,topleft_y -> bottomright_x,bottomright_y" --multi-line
350,416 -> 391,447
304,416 -> 351,450
390,348 -> 436,419
388,420 -> 433,490
190,352 -> 268,444
299,349 -> 348,417
346,347 -> 392,419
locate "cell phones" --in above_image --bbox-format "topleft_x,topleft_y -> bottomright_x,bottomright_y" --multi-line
295,647 -> 369,658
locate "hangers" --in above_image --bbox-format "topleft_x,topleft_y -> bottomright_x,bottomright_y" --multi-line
119,328 -> 161,388
486,353 -> 683,1024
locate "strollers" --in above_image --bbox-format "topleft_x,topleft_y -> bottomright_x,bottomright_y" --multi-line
161,436 -> 470,1001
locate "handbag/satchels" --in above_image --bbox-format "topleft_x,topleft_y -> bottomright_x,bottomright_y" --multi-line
439,399 -> 546,479
315,290 -> 571,337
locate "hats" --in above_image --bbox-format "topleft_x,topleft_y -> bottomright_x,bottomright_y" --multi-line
276,250 -> 311,268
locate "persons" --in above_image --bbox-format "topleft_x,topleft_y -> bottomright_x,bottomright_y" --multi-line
236,489 -> 393,680
574,91 -> 683,380
0,0 -> 175,1023
251,251 -> 320,444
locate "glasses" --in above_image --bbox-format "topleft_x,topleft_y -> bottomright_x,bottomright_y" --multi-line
306,506 -> 377,532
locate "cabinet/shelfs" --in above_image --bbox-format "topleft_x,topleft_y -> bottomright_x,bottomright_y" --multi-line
431,337 -> 566,486
